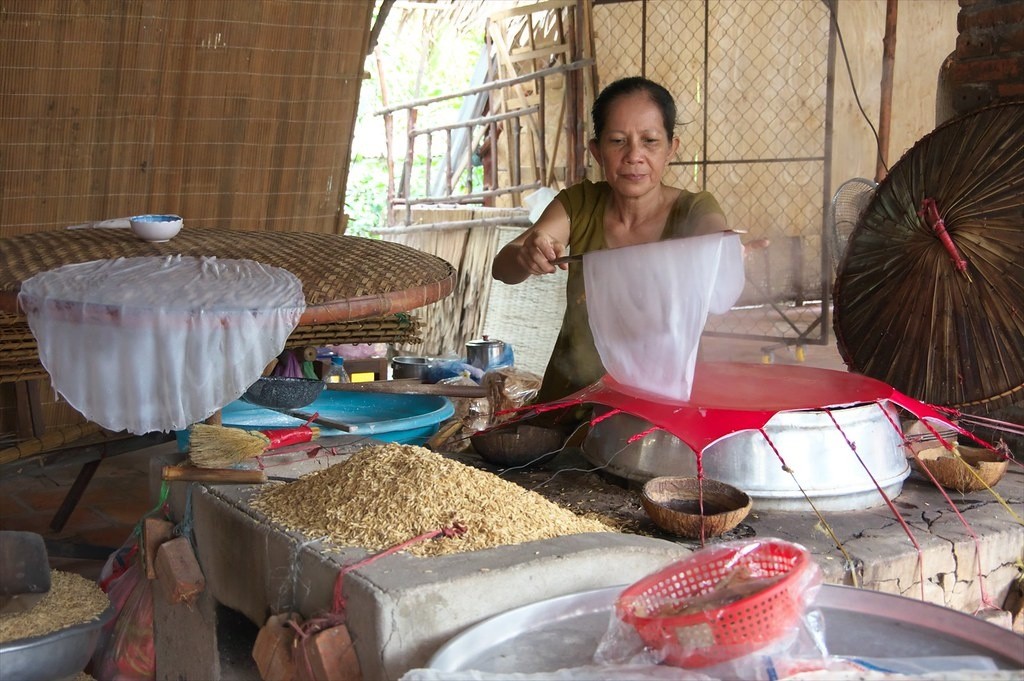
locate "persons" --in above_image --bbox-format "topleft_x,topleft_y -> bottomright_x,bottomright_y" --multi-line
492,76 -> 731,442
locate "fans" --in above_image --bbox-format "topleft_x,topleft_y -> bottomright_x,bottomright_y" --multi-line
826,177 -> 880,274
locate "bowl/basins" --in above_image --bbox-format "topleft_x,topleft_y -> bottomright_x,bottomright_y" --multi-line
917,445 -> 1010,493
175,390 -> 455,455
0,602 -> 119,681
471,428 -> 562,467
241,375 -> 325,412
129,214 -> 183,243
641,476 -> 753,539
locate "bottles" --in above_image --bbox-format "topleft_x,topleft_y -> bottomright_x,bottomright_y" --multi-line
321,356 -> 350,383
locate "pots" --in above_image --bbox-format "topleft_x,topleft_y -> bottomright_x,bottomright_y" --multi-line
392,356 -> 434,379
582,360 -> 912,513
466,334 -> 505,371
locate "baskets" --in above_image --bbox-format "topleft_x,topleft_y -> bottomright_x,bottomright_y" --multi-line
616,537 -> 809,668
